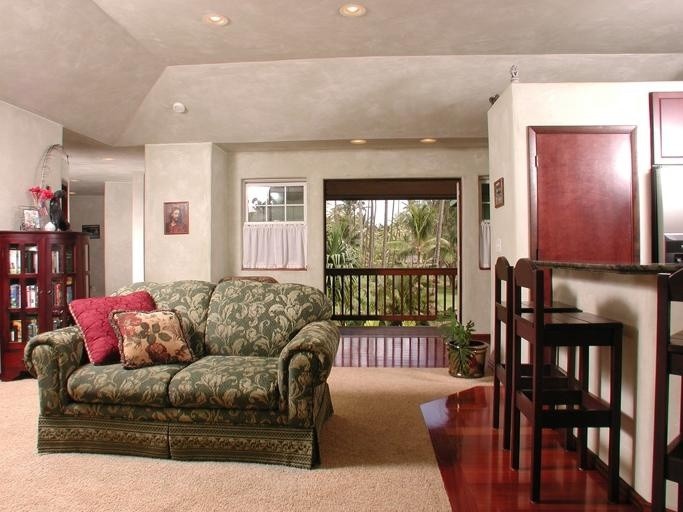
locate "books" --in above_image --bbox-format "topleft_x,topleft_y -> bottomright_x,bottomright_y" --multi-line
5,243 -> 76,343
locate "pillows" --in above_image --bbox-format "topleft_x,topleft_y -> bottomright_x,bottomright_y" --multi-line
66,290 -> 196,368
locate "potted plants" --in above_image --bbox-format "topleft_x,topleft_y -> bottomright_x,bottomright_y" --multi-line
436,306 -> 488,380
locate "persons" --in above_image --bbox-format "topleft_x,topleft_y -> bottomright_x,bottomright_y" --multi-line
164,206 -> 187,234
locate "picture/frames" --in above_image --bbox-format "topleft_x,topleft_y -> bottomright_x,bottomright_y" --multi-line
19,205 -> 42,232
81,224 -> 100,239
163,203 -> 189,235
493,177 -> 504,208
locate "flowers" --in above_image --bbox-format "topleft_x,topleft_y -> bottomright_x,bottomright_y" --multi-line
27,185 -> 53,210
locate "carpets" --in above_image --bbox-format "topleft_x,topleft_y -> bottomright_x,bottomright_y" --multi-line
1,366 -> 501,512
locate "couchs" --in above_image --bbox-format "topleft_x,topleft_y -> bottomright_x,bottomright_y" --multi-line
21,280 -> 340,469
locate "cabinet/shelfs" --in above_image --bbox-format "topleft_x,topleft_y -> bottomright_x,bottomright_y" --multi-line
0,231 -> 90,381
